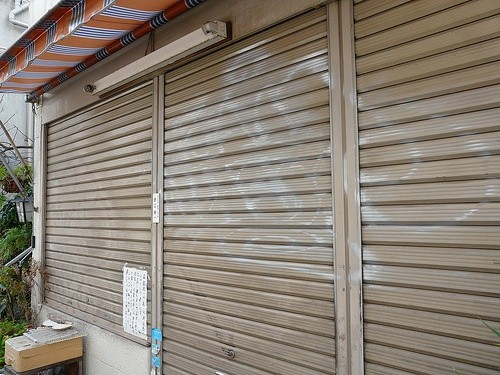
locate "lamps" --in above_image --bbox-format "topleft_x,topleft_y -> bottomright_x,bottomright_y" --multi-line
83,14 -> 227,99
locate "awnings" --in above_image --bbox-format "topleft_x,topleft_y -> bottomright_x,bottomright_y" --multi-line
0,0 -> 202,98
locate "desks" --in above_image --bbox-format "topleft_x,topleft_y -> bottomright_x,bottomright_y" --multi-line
3,356 -> 83,375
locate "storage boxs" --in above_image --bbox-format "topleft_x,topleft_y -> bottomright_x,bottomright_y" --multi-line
4,325 -> 85,372
